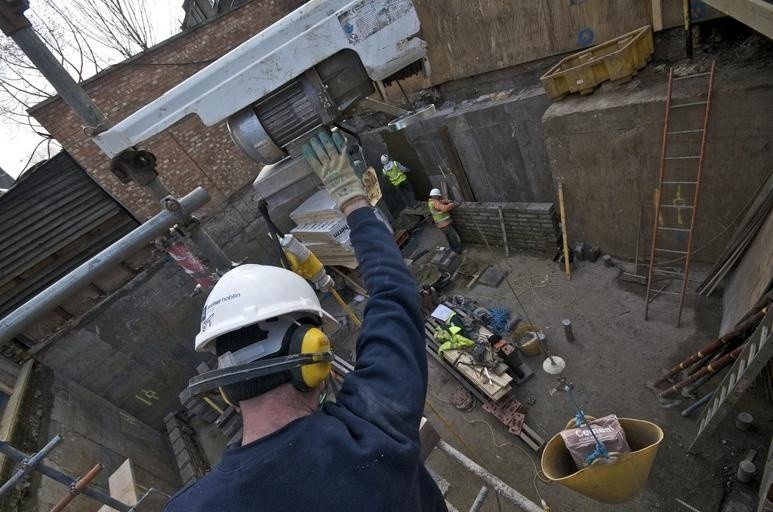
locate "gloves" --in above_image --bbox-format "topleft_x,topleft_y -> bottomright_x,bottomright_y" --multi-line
302,132 -> 368,212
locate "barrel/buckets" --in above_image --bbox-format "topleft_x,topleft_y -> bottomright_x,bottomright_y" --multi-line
541,414 -> 663,503
511,324 -> 542,357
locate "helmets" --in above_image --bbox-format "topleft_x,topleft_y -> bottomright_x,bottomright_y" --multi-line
381,154 -> 389,164
428,188 -> 442,197
192,263 -> 342,352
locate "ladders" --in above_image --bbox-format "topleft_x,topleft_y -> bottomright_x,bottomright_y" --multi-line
686,303 -> 773,456
644,59 -> 715,328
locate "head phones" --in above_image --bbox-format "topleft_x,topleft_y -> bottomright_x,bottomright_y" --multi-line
187,324 -> 333,394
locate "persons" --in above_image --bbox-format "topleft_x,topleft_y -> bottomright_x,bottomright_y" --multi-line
162,131 -> 447,511
427,187 -> 462,254
379,153 -> 422,209
